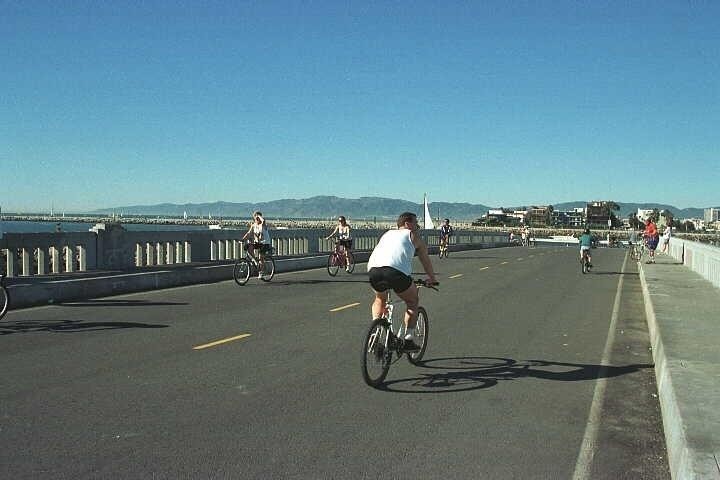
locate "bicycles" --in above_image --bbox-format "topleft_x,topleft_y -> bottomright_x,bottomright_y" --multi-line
327,239 -> 357,277
0,275 -> 10,320
360,280 -> 440,388
582,249 -> 590,274
232,241 -> 275,286
438,234 -> 449,259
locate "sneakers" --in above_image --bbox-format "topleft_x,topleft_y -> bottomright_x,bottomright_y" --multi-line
258,271 -> 264,279
403,338 -> 421,352
345,265 -> 351,271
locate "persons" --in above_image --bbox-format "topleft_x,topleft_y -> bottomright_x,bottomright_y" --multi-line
509,225 -> 532,246
642,216 -> 660,264
661,220 -> 671,254
326,215 -> 353,272
578,229 -> 596,266
367,212 -> 438,352
440,218 -> 454,248
240,210 -> 271,280
